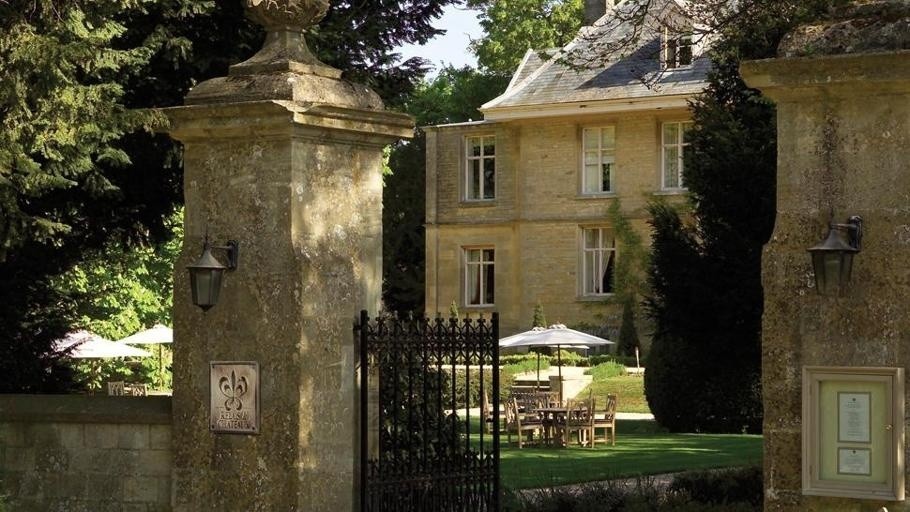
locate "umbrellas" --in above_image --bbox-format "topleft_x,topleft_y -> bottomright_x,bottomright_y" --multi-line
503,322 -> 615,407
499,327 -> 590,392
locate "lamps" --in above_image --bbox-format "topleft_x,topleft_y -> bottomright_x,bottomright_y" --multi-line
805,208 -> 862,302
184,222 -> 238,316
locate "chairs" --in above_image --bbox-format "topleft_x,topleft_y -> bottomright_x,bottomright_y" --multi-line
131,384 -> 148,398
482,376 -> 618,450
107,380 -> 125,397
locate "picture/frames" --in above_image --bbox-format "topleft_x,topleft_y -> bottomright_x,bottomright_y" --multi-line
801,365 -> 905,502
209,362 -> 261,437
